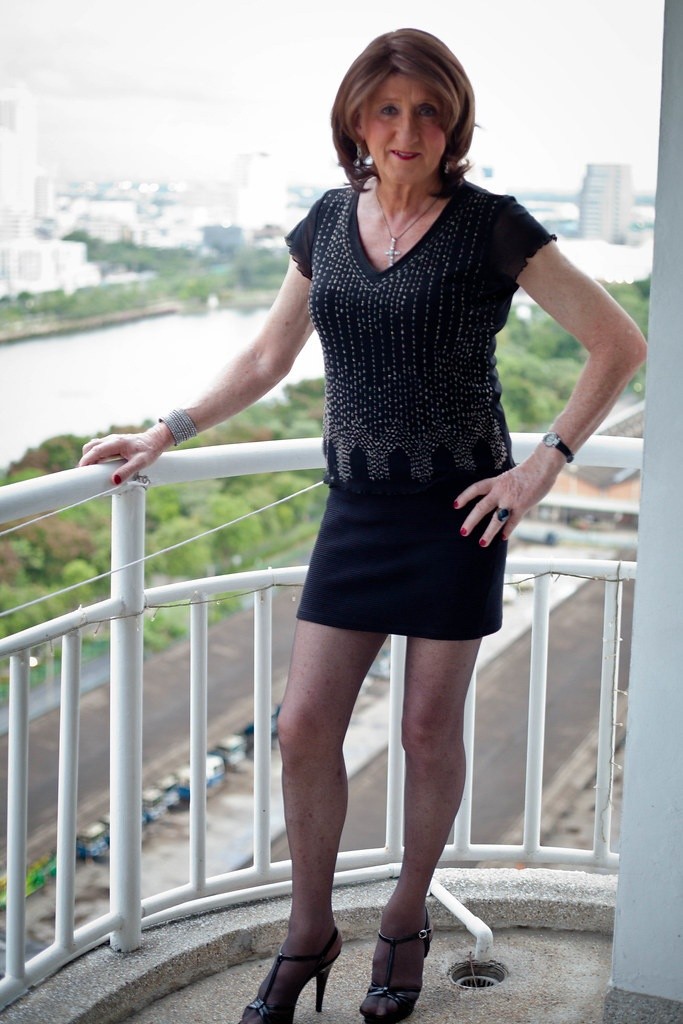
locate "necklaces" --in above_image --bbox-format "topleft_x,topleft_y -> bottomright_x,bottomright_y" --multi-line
375,182 -> 457,268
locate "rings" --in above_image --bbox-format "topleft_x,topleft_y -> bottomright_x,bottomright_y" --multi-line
498,507 -> 514,524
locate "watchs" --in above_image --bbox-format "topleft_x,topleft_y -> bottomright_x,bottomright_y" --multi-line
543,432 -> 574,464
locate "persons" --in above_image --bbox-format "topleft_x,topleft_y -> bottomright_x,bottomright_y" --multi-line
77,27 -> 647,1024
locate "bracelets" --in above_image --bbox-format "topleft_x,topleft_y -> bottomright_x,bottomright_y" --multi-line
157,409 -> 197,444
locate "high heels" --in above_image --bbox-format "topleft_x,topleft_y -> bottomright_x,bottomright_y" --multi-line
246,927 -> 341,1024
359,907 -> 431,1024
